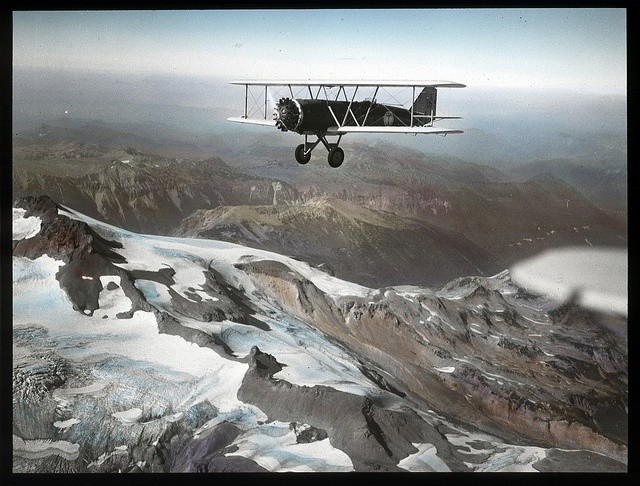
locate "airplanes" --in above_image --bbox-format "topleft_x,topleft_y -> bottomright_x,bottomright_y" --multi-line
226,78 -> 467,167
